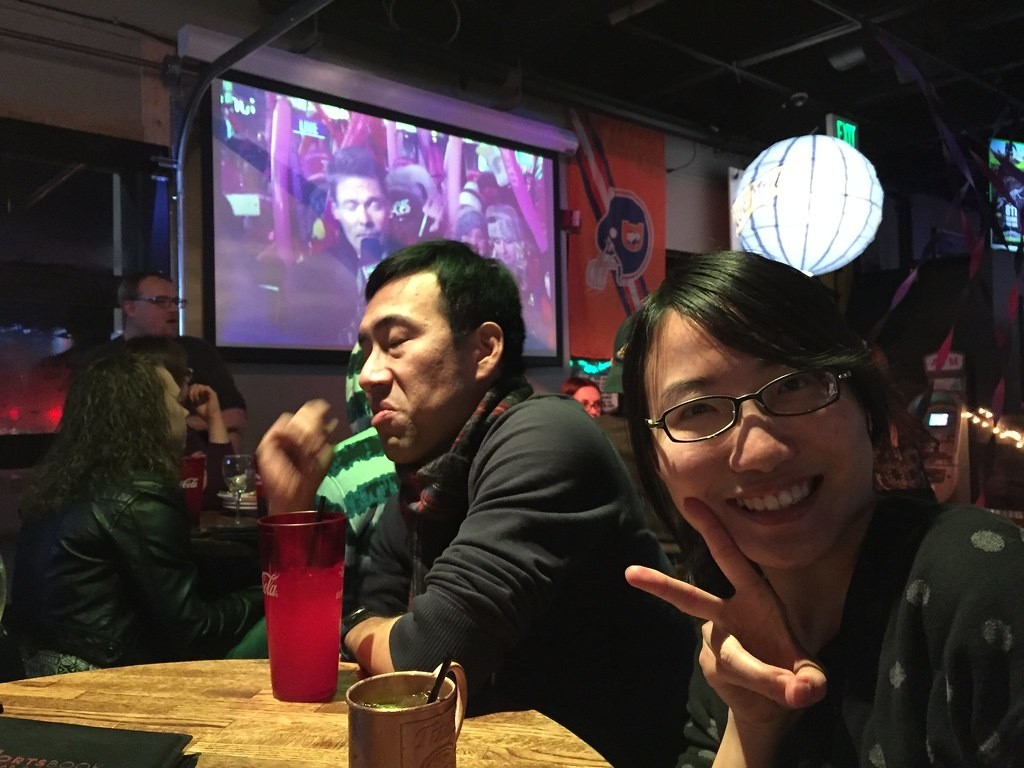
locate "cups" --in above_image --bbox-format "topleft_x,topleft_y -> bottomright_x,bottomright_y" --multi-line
346,662 -> 467,768
258,510 -> 347,701
178,454 -> 205,528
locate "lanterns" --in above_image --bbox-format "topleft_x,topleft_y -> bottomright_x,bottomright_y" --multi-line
732,136 -> 885,278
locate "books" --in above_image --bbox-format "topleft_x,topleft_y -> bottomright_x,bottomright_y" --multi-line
0,717 -> 193,768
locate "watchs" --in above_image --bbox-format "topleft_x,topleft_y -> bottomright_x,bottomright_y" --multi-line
339,605 -> 388,662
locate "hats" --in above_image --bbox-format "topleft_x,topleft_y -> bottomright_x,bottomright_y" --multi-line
458,180 -> 483,212
384,163 -> 444,237
456,211 -> 486,236
485,203 -> 524,240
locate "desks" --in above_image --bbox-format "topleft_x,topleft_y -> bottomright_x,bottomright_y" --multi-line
0,660 -> 614,768
190,509 -> 259,557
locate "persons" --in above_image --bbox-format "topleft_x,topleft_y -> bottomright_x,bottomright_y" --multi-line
621,250 -> 1024,768
255,237 -> 689,768
0,269 -> 269,681
212,78 -> 559,354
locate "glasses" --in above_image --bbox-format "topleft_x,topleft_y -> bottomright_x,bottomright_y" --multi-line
180,367 -> 194,383
127,295 -> 188,309
580,399 -> 605,413
644,365 -> 855,444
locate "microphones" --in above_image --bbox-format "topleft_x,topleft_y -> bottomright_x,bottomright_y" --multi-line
358,238 -> 381,283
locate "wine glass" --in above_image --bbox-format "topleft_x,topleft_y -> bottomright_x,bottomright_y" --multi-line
223,455 -> 255,526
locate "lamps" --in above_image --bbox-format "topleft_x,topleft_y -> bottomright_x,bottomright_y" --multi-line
732,136 -> 885,276
